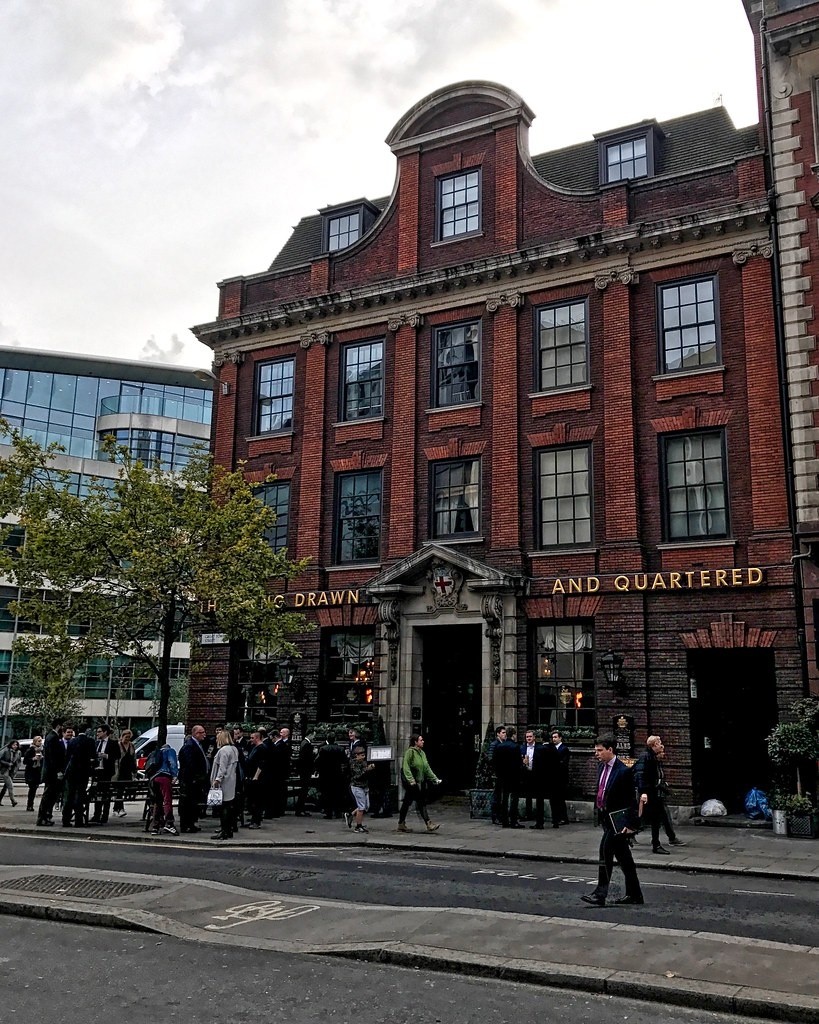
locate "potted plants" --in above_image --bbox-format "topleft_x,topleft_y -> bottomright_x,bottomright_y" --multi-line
469,716 -> 496,820
764,722 -> 816,838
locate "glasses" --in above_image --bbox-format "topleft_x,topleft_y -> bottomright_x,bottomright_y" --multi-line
196,732 -> 204,734
97,731 -> 102,733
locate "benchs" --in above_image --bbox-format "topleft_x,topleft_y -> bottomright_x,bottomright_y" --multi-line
71,781 -> 155,833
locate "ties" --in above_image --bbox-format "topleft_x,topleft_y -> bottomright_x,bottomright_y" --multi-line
98,740 -> 104,752
597,763 -> 610,809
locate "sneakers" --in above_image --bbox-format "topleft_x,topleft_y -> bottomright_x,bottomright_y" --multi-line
345,813 -> 354,831
354,826 -> 369,834
397,821 -> 413,833
151,829 -> 161,834
427,820 -> 440,831
163,824 -> 179,835
668,838 -> 686,846
630,838 -> 640,846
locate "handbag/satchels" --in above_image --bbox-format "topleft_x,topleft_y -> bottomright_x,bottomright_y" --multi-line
400,750 -> 419,785
635,779 -> 672,803
207,781 -> 223,805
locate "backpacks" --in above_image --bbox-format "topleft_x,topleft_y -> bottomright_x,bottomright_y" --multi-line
142,746 -> 165,777
118,742 -> 136,776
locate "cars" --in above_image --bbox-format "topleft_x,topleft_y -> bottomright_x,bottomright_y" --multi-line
132,721 -> 185,776
0,738 -> 46,783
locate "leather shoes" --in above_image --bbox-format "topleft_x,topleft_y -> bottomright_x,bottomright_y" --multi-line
511,823 -> 525,828
615,894 -> 644,904
529,825 -> 544,829
559,821 -> 570,825
215,828 -> 221,833
652,846 -> 670,854
580,894 -> 605,906
503,823 -> 511,827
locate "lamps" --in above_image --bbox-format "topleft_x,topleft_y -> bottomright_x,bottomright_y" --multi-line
600,648 -> 625,688
192,369 -> 229,395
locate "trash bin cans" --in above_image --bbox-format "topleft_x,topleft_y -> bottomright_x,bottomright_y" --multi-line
772,809 -> 787,835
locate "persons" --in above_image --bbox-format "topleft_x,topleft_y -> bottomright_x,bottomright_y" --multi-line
22,735 -> 44,811
0,740 -> 21,807
625,735 -> 670,855
487,726 -> 573,830
628,747 -> 687,847
36,717 -> 376,841
581,735 -> 644,906
398,733 -> 442,833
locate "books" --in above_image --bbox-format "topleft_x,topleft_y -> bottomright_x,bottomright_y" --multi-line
608,808 -> 628,834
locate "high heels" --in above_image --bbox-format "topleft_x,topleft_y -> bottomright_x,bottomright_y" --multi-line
228,827 -> 233,838
211,832 -> 228,840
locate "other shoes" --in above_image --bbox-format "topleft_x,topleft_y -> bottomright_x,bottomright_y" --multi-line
27,806 -> 34,811
12,802 -> 17,807
118,809 -> 127,817
37,820 -> 54,826
113,811 -> 118,816
